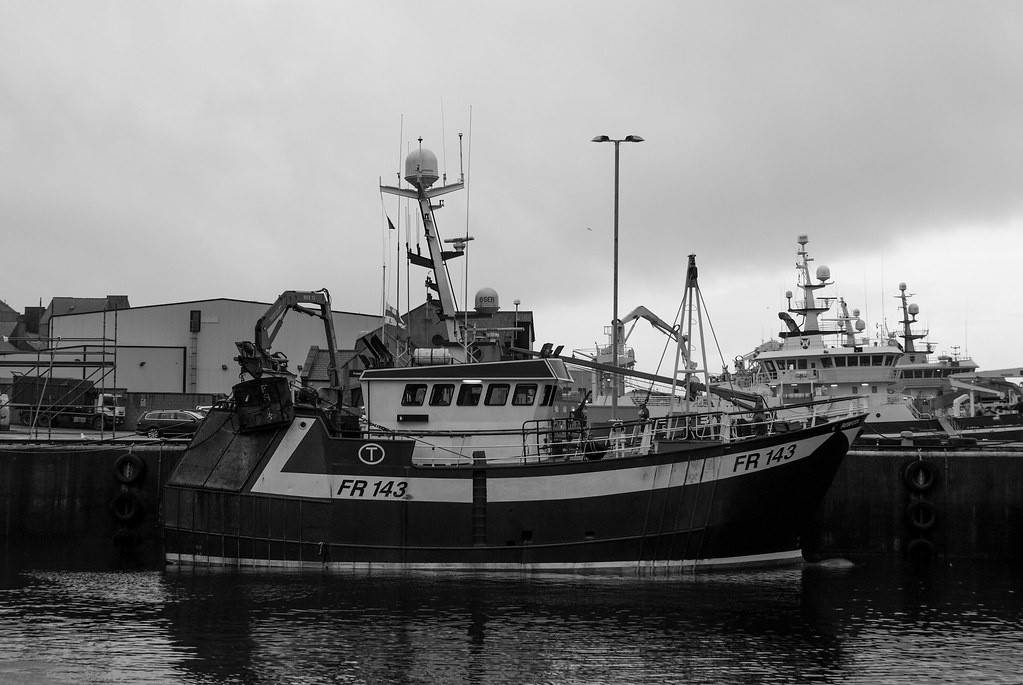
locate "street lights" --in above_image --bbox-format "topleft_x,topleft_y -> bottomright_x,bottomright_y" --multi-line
590,135 -> 645,419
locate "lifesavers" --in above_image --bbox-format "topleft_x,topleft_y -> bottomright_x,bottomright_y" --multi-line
906,538 -> 937,556
903,460 -> 938,491
905,499 -> 939,531
111,529 -> 141,553
113,454 -> 146,482
109,491 -> 137,520
582,443 -> 606,460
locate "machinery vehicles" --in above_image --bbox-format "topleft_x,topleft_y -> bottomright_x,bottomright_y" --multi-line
233,288 -> 365,434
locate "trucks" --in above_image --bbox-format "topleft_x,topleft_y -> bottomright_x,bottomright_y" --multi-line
8,370 -> 126,431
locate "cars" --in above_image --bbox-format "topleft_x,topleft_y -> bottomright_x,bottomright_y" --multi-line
134,408 -> 206,438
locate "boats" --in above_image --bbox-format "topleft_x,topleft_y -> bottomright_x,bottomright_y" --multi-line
155,102 -> 1022,574
